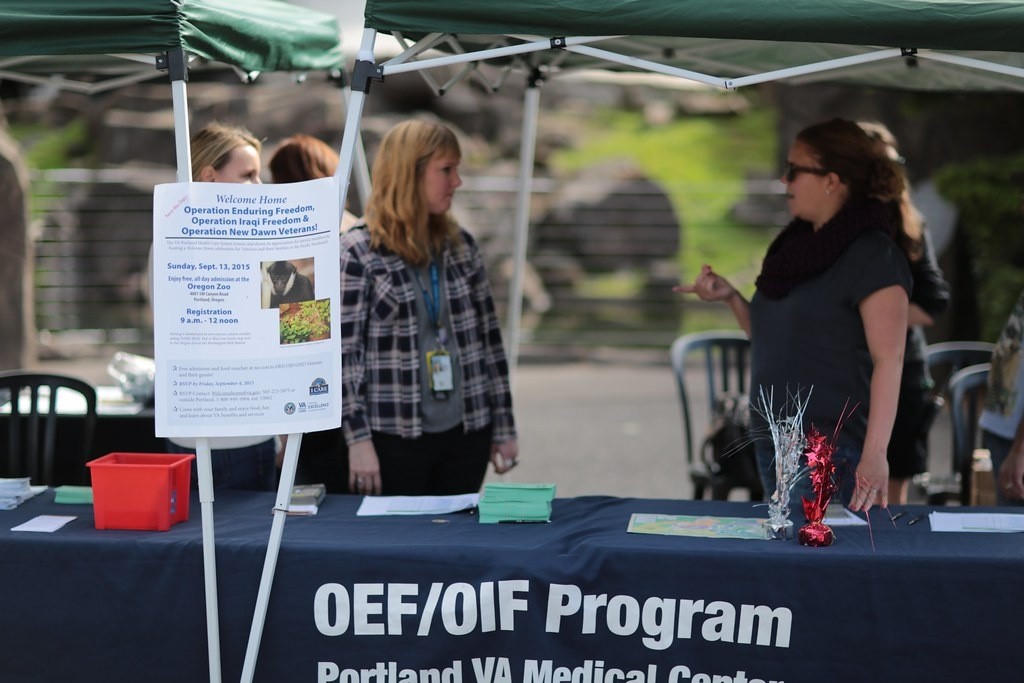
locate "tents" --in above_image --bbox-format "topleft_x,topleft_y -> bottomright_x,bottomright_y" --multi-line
0,0 -> 337,683
242,0 -> 1024,683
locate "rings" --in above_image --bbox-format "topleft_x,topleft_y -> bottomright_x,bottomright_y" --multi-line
872,487 -> 878,493
504,459 -> 513,467
357,480 -> 361,484
512,456 -> 521,465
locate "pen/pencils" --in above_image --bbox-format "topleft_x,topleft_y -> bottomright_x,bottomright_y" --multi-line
890,510 -> 926,526
496,520 -> 552,524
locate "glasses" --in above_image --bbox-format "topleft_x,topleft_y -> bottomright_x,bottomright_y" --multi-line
786,160 -> 845,185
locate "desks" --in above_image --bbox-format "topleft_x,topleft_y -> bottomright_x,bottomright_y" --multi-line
0,384 -> 1024,683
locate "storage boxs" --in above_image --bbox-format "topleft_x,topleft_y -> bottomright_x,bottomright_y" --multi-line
85,452 -> 197,532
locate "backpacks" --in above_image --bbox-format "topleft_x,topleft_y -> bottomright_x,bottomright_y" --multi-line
701,425 -> 760,488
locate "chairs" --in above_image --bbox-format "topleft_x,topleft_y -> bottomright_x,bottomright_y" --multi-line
919,339 -> 996,509
671,329 -> 762,502
0,373 -> 97,486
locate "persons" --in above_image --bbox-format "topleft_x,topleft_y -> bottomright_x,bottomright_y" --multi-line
148,120 -> 277,495
978,286 -> 1024,507
673,118 -> 922,512
855,120 -> 954,504
339,120 -> 520,495
269,131 -> 359,493
